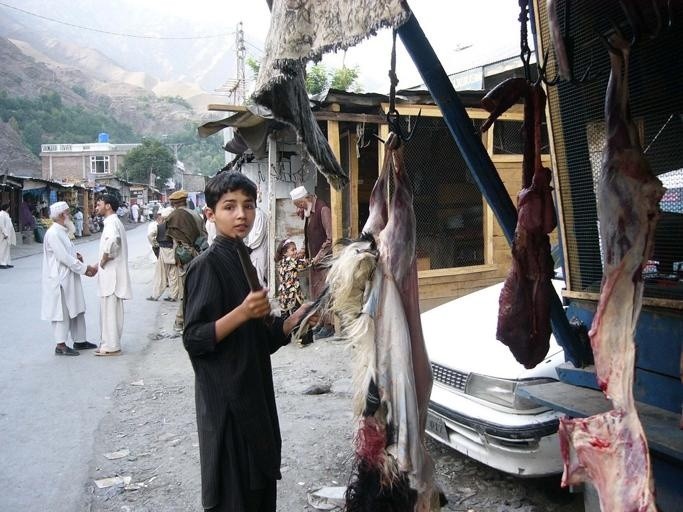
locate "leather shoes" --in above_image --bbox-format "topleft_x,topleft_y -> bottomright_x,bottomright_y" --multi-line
315,326 -> 335,338
312,322 -> 322,334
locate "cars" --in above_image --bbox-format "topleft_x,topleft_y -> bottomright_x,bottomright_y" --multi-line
142,200 -> 165,218
419,266 -> 564,481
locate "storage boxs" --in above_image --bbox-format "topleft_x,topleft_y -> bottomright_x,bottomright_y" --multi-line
437,183 -> 480,204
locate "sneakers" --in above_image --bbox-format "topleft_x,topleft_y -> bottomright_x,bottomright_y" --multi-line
146,296 -> 175,301
56,341 -> 120,356
1,263 -> 13,270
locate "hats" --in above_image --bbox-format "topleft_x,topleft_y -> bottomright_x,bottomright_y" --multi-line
169,190 -> 189,203
50,201 -> 68,214
290,185 -> 307,199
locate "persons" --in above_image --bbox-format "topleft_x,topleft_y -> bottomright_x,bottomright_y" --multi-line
1,202 -> 16,271
43,201 -> 97,356
290,186 -> 332,338
131,190 -> 269,331
74,209 -> 83,238
179,173 -> 315,512
274,239 -> 315,344
87,195 -> 129,356
